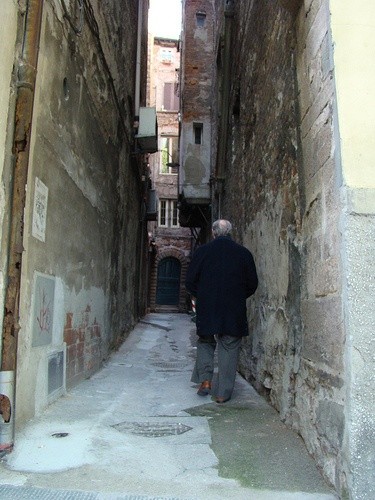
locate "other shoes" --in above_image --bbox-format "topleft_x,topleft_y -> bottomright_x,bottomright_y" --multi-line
197,380 -> 211,396
216,397 -> 228,403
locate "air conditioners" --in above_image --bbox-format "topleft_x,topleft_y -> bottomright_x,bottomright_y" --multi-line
138,107 -> 158,153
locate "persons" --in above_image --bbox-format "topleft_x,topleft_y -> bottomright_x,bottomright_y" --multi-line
185,219 -> 259,403
